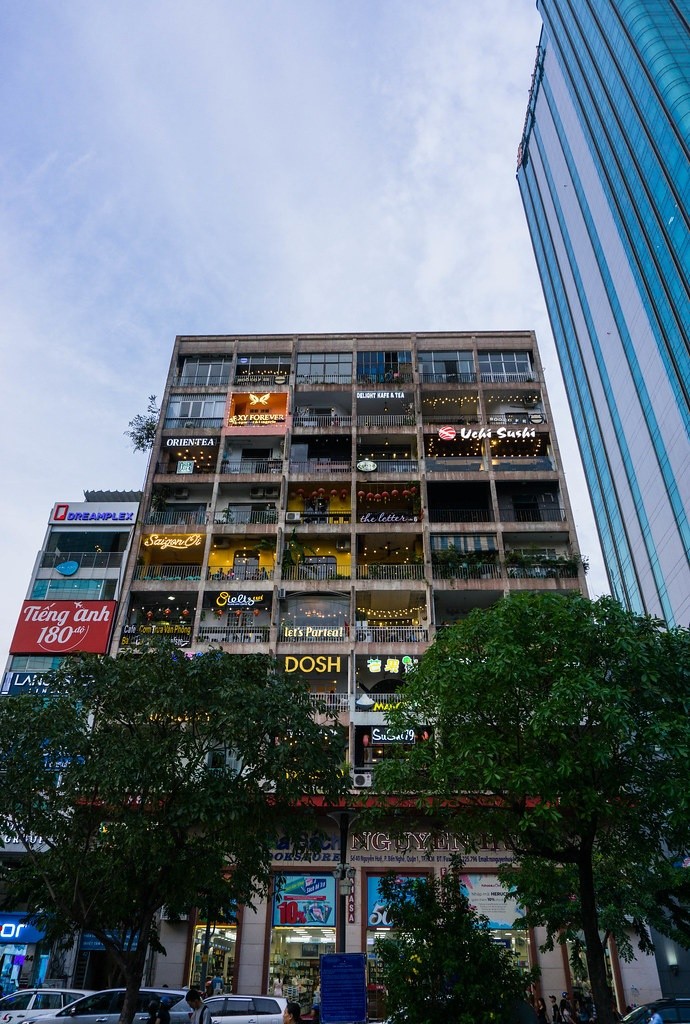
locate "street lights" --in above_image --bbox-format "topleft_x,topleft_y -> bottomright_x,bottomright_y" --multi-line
326,812 -> 363,951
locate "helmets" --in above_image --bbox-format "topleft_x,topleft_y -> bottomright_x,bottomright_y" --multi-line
205,980 -> 212,987
562,991 -> 569,998
160,998 -> 173,1006
148,1000 -> 159,1009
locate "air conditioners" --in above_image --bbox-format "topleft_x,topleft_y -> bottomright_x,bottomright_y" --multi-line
285,512 -> 300,523
257,781 -> 277,793
353,774 -> 371,787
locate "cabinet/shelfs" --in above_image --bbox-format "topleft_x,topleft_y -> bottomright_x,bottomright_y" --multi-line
191,939 -> 387,1016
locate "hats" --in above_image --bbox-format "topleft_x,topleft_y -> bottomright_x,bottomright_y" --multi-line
549,995 -> 556,999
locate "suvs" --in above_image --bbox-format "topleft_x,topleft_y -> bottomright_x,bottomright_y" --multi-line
17,987 -> 195,1024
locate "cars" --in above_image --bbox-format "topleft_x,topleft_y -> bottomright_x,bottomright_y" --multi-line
624,997 -> 690,1023
0,988 -> 105,1024
202,995 -> 289,1024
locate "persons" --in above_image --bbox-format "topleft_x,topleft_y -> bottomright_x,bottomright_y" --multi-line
217,568 -> 225,580
332,414 -> 340,426
252,569 -> 260,580
260,568 -> 268,580
186,990 -> 211,1024
148,1000 -> 170,1024
273,973 -> 282,996
648,1008 -> 663,1024
227,569 -> 234,580
282,1003 -> 301,1024
211,972 -> 223,989
538,990 -> 598,1024
207,566 -> 211,580
385,370 -> 393,383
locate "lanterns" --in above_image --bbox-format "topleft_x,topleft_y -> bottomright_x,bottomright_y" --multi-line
358,487 -> 417,504
296,488 -> 347,498
